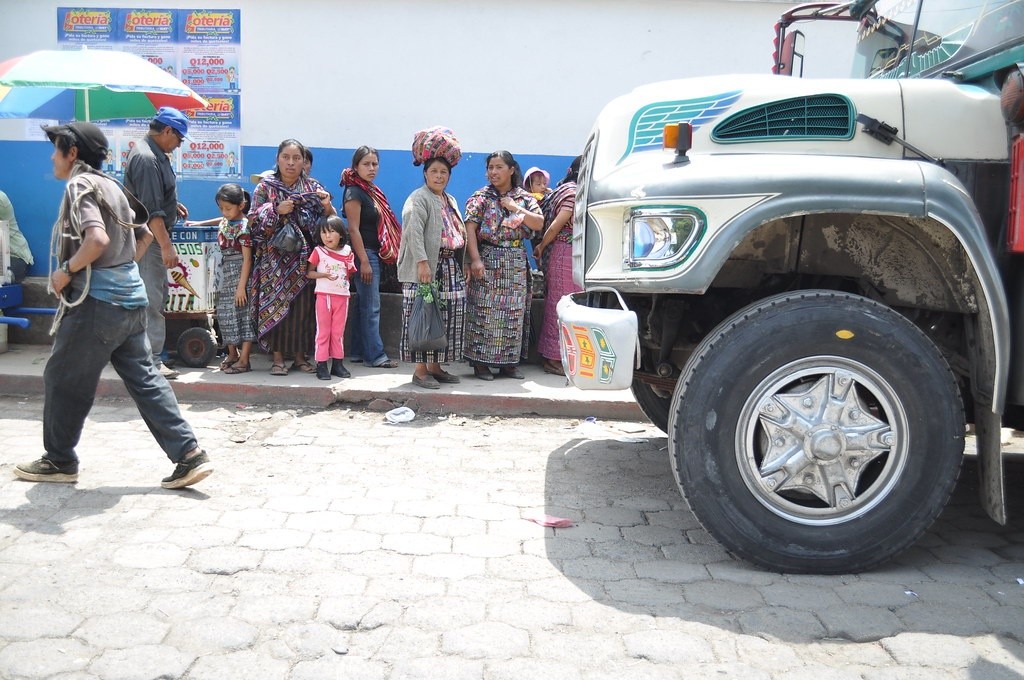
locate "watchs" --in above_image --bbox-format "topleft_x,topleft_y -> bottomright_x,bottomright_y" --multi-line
62,261 -> 74,276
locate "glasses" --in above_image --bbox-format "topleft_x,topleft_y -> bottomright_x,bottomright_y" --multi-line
172,131 -> 185,144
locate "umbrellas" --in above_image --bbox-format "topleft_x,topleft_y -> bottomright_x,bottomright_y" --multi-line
0,45 -> 209,123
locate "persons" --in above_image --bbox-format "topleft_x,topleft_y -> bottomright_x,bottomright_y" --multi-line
532,155 -> 583,376
342,145 -> 397,368
246,139 -> 337,375
125,106 -> 188,379
307,215 -> 357,381
0,191 -> 34,281
14,121 -> 215,489
396,156 -> 470,389
523,166 -> 553,205
304,148 -> 313,175
464,150 -> 544,381
184,184 -> 257,374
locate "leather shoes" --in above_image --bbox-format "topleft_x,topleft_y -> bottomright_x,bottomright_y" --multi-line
316,361 -> 332,380
330,358 -> 352,378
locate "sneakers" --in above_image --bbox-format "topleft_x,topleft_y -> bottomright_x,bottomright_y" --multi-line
162,449 -> 214,490
14,457 -> 80,483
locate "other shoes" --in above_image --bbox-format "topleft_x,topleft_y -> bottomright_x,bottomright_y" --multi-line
429,370 -> 461,384
500,365 -> 525,379
473,364 -> 494,380
544,360 -> 565,376
412,373 -> 440,389
377,360 -> 398,368
163,359 -> 176,366
155,363 -> 180,380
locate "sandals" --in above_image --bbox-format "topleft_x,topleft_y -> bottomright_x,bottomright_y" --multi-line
219,357 -> 239,370
224,361 -> 251,374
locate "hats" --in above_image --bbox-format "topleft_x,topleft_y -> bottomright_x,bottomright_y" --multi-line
154,107 -> 197,146
42,121 -> 109,159
251,170 -> 276,183
523,167 -> 550,190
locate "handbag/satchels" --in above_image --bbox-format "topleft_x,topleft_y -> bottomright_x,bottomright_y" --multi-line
272,218 -> 303,253
408,282 -> 448,352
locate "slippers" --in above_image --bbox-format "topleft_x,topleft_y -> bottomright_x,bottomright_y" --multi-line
271,364 -> 289,374
291,361 -> 316,373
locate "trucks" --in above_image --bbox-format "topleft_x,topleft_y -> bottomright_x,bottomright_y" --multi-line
556,0 -> 1024,576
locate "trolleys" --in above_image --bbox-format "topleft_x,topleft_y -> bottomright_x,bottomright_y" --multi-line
0,281 -> 57,330
162,222 -> 222,368
